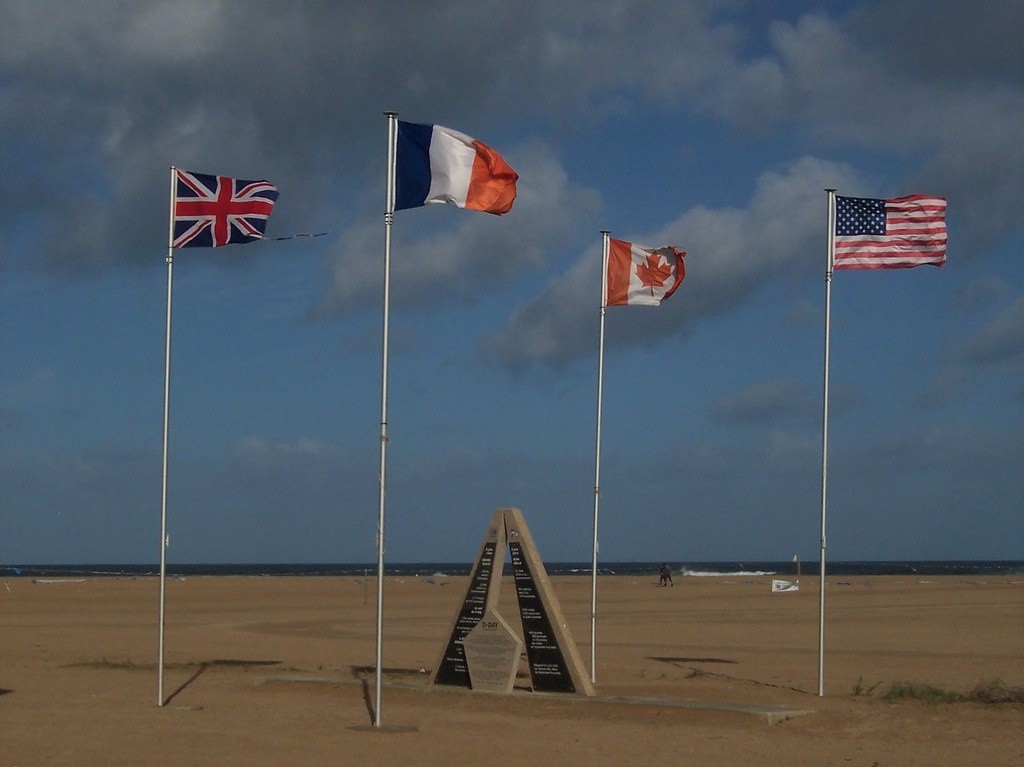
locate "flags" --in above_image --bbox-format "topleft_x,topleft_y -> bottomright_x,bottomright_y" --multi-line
173,168 -> 281,248
831,193 -> 947,271
606,235 -> 686,306
393,119 -> 519,216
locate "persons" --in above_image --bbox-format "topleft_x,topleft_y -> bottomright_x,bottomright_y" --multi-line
659,563 -> 674,588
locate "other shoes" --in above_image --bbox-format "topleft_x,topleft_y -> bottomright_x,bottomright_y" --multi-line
664,584 -> 666,586
671,584 -> 673,587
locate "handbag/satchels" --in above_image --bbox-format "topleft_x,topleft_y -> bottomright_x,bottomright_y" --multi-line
661,572 -> 663,575
